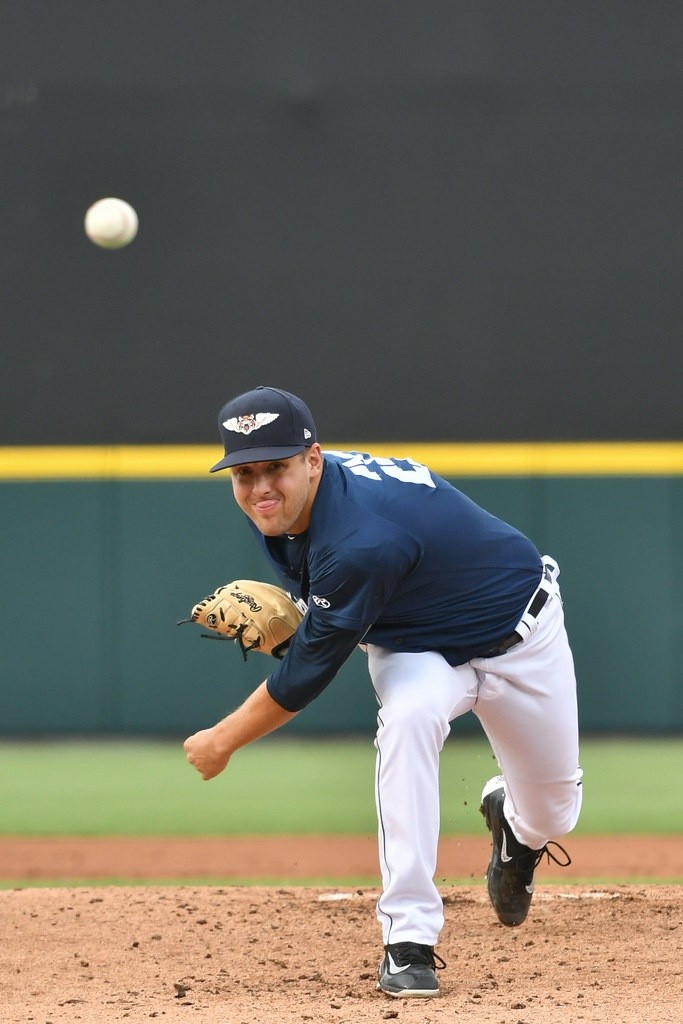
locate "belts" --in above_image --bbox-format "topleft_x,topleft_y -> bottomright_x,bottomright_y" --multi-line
474,565 -> 552,657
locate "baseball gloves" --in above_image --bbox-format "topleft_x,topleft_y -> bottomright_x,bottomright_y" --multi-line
190,579 -> 309,662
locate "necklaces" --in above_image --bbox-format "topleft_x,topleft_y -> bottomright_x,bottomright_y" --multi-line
286,533 -> 297,540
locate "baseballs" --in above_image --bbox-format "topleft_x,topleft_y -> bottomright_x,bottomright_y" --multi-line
83,196 -> 139,252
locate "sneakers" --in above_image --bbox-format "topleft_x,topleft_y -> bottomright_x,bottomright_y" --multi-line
479,775 -> 572,928
376,942 -> 446,998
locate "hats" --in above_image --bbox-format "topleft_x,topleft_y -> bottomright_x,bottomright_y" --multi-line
209,385 -> 317,473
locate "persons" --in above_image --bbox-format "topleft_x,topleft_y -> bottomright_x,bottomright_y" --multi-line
182,386 -> 587,999
